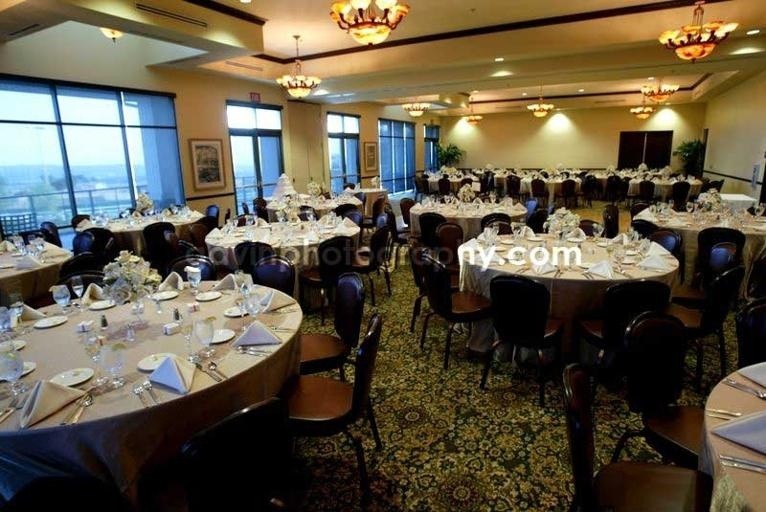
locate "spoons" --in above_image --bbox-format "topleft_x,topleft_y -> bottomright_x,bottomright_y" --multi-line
70,395 -> 92,425
725,376 -> 766,399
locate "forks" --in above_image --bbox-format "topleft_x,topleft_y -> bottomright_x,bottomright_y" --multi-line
133,381 -> 163,412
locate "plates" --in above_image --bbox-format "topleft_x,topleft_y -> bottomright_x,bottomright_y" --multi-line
0,291 -> 250,386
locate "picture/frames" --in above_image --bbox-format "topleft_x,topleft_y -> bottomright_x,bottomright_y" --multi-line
364,142 -> 378,171
189,138 -> 227,191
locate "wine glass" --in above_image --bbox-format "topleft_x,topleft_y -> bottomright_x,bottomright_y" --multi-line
54,274 -> 85,314
234,272 -> 261,332
187,266 -> 202,294
0,232 -> 50,269
89,166 -> 766,260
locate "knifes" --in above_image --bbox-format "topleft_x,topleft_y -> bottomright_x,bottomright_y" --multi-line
0,392 -> 30,425
513,264 -> 635,281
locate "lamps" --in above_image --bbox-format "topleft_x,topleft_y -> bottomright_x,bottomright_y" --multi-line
276,35 -> 321,100
329,0 -> 410,45
656,0 -> 738,62
100,26 -> 127,45
401,78 -> 679,121
